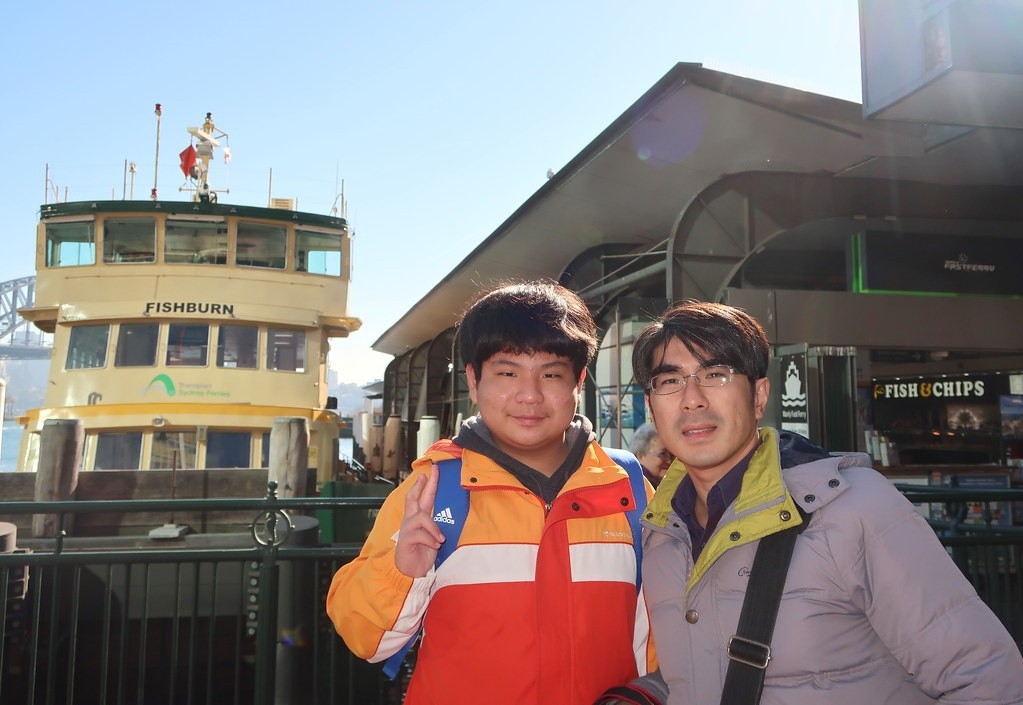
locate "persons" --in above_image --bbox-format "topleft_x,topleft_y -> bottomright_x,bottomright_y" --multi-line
627,423 -> 676,491
326,277 -> 658,705
592,296 -> 1023,705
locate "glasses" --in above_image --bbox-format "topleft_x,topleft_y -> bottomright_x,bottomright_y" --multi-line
645,447 -> 676,462
644,364 -> 750,394
